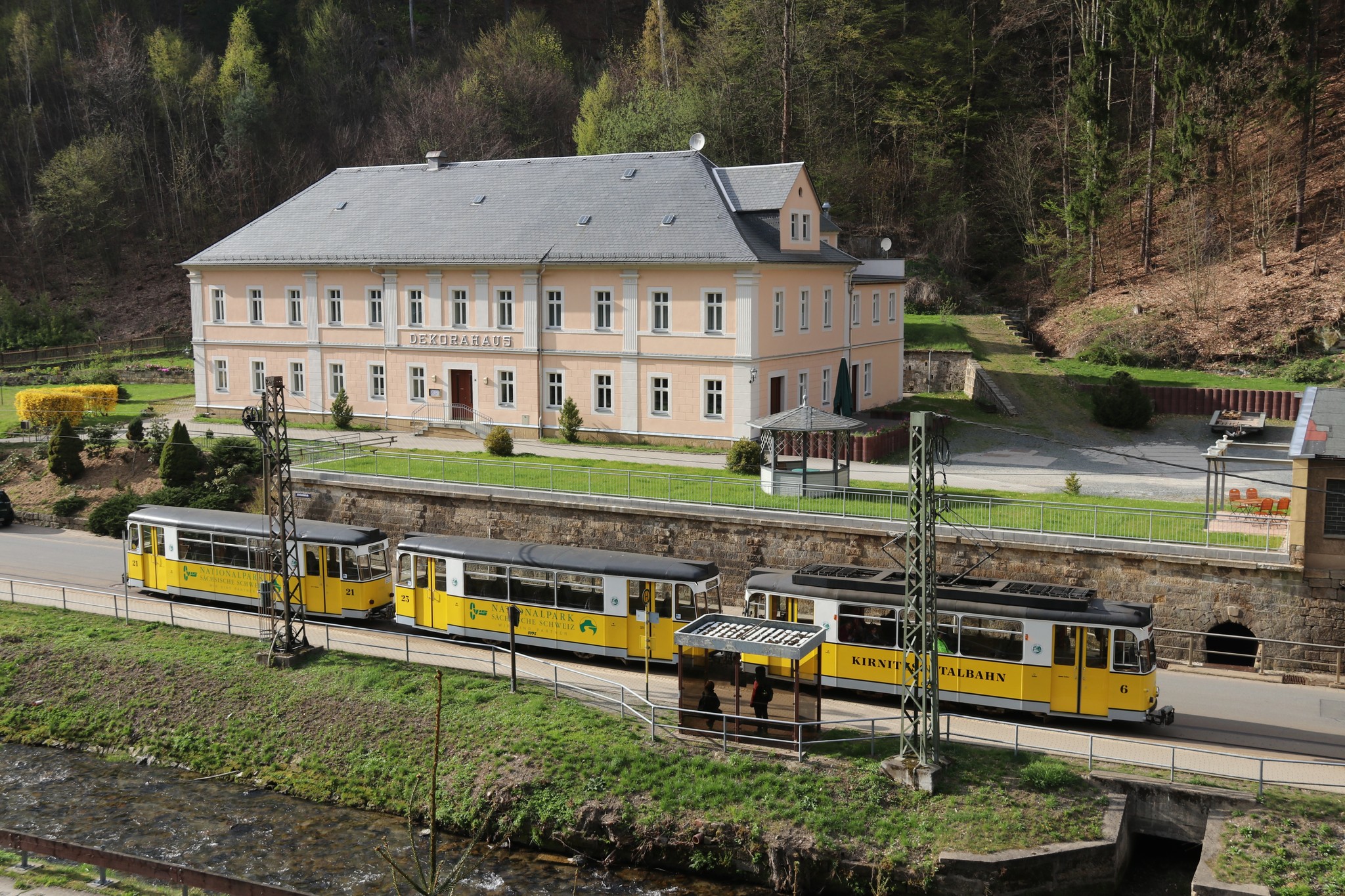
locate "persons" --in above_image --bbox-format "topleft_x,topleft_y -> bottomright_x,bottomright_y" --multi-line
1055,635 -> 1071,665
1087,647 -> 1107,669
750,666 -> 768,735
702,681 -> 722,729
348,558 -> 354,567
931,622 -> 954,654
306,551 -> 320,576
184,541 -> 212,562
661,592 -> 671,618
1115,638 -> 1129,663
839,610 -> 903,647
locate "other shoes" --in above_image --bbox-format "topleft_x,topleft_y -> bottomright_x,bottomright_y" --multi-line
706,720 -> 715,731
753,726 -> 768,736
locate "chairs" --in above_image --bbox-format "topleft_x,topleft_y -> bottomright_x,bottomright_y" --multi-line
342,561 -> 356,579
306,551 -> 317,574
195,549 -> 217,564
225,544 -> 254,567
465,574 -> 575,608
1229,488 -> 1290,529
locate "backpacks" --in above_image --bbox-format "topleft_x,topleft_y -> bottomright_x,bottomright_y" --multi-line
759,685 -> 774,702
697,691 -> 714,712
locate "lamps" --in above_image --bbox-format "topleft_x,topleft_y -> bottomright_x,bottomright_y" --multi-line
749,365 -> 757,384
183,345 -> 196,362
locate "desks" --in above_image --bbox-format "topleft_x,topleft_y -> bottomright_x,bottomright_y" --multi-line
1234,498 -> 1279,526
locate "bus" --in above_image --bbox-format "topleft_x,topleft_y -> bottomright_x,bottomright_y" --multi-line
121,504 -> 396,622
740,562 -> 1175,725
394,531 -> 723,668
